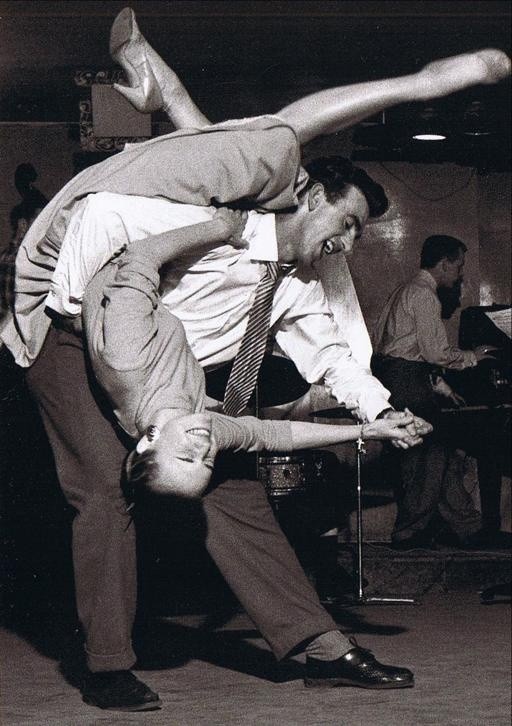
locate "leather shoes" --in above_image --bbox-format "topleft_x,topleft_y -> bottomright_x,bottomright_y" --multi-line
82,665 -> 163,712
423,47 -> 512,97
439,528 -> 502,547
303,645 -> 415,689
394,514 -> 445,552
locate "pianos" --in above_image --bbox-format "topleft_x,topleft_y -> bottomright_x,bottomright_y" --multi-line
426,305 -> 509,598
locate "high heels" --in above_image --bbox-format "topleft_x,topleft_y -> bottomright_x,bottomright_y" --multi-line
108,7 -> 166,116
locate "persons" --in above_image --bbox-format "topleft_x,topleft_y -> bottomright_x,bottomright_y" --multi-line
1,3 -> 511,515
371,229 -> 501,551
0,195 -> 49,320
0,153 -> 416,712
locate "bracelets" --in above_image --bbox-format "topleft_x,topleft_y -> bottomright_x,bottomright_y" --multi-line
356,422 -> 367,453
376,406 -> 392,421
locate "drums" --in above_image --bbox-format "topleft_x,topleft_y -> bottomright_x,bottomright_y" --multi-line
257,457 -> 323,498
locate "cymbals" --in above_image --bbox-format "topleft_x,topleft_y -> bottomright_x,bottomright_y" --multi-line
204,356 -> 310,409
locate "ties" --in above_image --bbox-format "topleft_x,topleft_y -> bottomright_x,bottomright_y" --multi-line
221,261 -> 296,418
